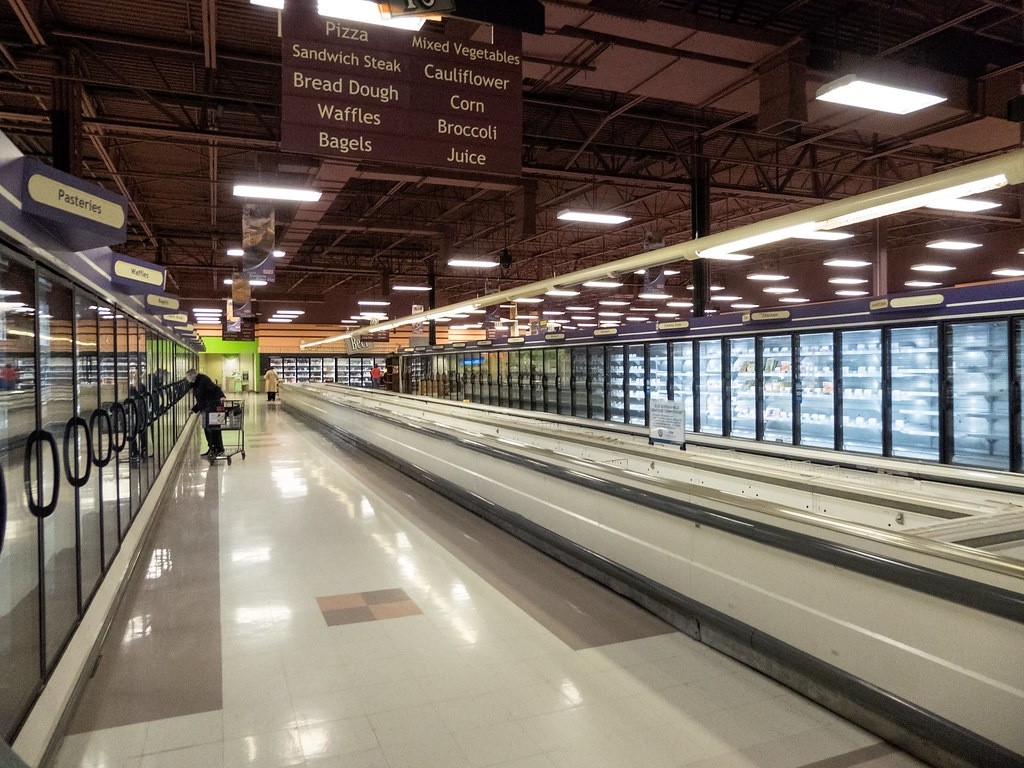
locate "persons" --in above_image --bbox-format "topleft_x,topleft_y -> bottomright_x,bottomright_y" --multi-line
128,368 -> 169,457
185,369 -> 226,458
5,364 -> 16,390
264,366 -> 278,401
371,364 -> 380,389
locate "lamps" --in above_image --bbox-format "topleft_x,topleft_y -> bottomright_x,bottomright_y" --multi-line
192,0 -> 1024,351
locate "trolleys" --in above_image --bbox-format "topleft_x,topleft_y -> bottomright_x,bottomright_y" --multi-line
206,399 -> 246,466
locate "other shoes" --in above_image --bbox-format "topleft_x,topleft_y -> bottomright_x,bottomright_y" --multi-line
201,448 -> 214,456
210,449 -> 224,459
273,399 -> 275,401
267,400 -> 271,401
